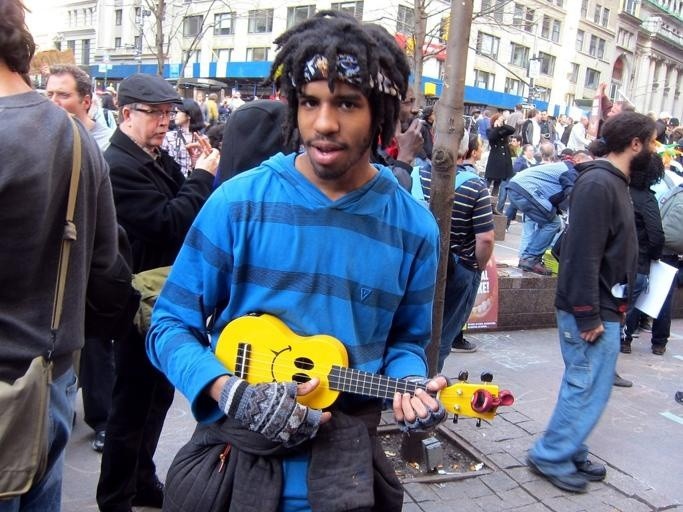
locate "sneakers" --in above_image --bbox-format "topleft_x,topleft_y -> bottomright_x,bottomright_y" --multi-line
618,338 -> 633,353
638,321 -> 654,333
574,458 -> 608,484
649,342 -> 668,355
518,253 -> 553,276
449,338 -> 478,353
522,450 -> 589,493
612,368 -> 635,388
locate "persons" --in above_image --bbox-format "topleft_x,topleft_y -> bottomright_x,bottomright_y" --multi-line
2,0 -> 683,512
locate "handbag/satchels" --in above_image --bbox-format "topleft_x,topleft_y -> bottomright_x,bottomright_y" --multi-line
0,352 -> 57,501
129,264 -> 173,335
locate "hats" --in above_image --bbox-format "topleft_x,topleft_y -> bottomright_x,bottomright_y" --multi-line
115,72 -> 184,107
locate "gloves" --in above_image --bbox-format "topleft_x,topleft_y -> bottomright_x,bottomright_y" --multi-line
213,372 -> 323,451
392,370 -> 455,434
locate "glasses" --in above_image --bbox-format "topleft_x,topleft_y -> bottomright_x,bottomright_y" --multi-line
129,106 -> 179,122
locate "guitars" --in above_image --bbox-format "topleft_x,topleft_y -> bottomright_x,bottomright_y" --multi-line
214,310 -> 514,428
